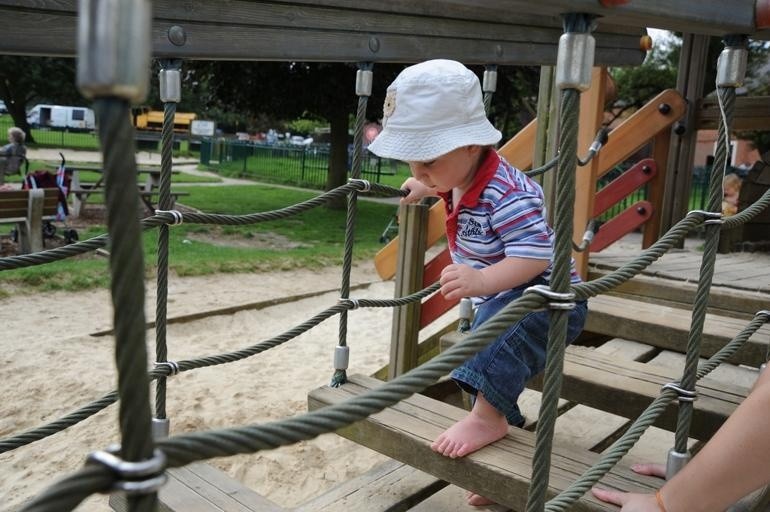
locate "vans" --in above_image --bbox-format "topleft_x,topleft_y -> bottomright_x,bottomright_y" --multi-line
291,135 -> 305,147
26,103 -> 96,134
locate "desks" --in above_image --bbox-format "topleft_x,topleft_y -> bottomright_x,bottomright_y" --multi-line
44,162 -> 182,218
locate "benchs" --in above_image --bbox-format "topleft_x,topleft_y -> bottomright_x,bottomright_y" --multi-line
69,189 -> 191,219
78,181 -> 148,194
0,185 -> 66,256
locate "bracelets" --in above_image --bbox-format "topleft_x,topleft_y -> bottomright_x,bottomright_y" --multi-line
653,491 -> 668,510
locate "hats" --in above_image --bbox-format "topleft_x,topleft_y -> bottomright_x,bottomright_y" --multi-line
367,60 -> 503,163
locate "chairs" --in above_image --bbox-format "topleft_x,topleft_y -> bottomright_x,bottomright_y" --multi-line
0,153 -> 31,182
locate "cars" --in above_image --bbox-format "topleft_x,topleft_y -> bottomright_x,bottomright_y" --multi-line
0,100 -> 14,114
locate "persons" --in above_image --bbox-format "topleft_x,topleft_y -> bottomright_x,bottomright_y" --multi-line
364,56 -> 589,509
588,356 -> 770,511
0,126 -> 28,183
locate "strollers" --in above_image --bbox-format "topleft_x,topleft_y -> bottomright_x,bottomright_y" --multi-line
11,152 -> 79,245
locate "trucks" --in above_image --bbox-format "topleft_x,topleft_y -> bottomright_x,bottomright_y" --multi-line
130,105 -> 199,151
